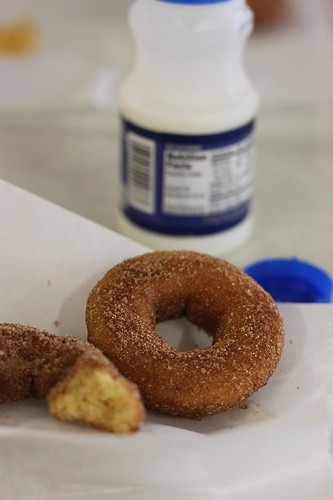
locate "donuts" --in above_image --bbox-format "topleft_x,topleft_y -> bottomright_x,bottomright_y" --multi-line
0,321 -> 145,434
86,251 -> 285,416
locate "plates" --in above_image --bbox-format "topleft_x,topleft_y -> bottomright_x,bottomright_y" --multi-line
1,175 -> 333,500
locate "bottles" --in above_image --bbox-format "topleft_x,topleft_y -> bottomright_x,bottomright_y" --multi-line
110,0 -> 265,260
